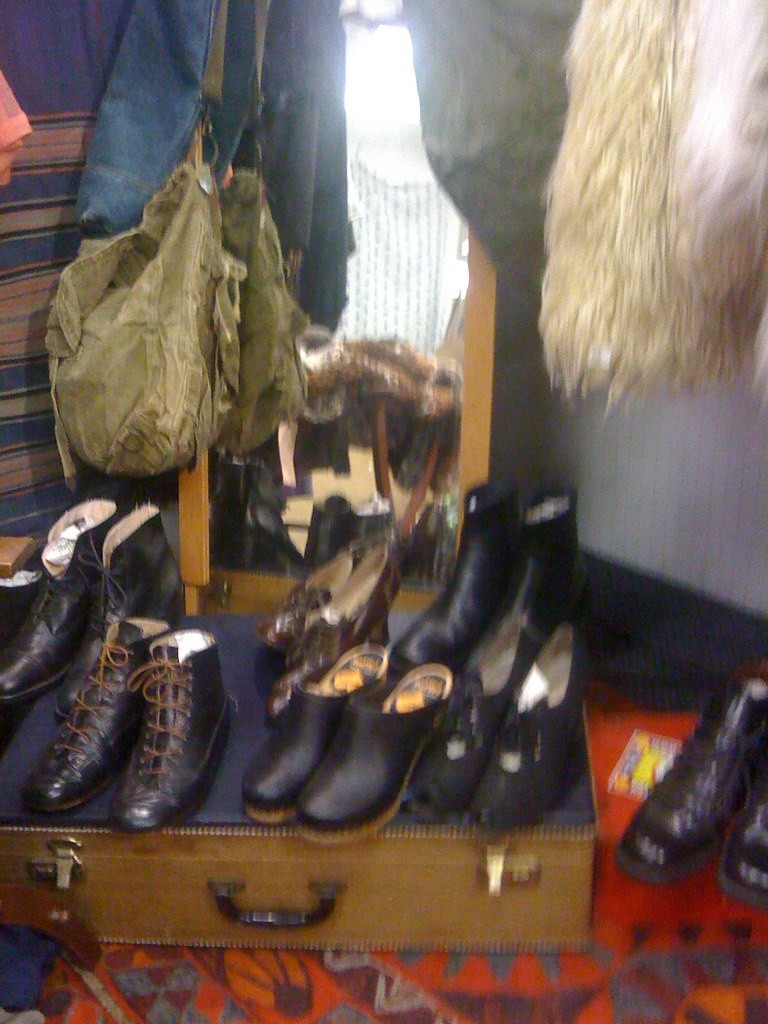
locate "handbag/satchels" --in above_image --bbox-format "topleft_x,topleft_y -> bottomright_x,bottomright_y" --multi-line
219,1 -> 307,454
43,0 -> 246,473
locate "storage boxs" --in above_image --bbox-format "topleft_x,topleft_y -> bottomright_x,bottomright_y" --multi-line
581,709 -> 768,948
0,613 -> 595,955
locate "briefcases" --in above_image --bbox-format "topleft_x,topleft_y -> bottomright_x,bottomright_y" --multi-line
0,612 -> 596,954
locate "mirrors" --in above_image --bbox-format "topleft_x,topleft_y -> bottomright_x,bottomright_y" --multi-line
182,0 -> 497,619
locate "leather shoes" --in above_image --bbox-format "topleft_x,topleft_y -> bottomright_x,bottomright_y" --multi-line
0,475 -> 767,907
